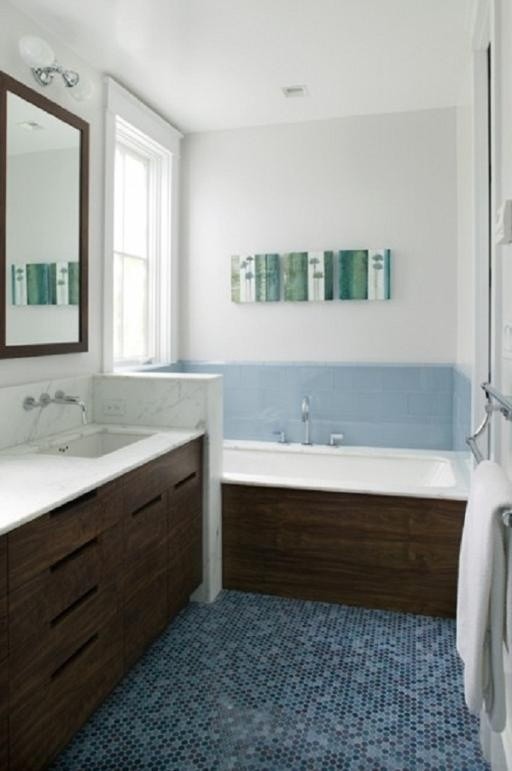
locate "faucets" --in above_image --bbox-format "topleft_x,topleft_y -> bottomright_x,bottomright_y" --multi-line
301,395 -> 311,443
42,393 -> 90,427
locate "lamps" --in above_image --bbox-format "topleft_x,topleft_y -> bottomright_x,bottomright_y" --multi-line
32,64 -> 79,87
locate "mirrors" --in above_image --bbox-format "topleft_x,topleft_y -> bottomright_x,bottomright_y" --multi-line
0,71 -> 89,358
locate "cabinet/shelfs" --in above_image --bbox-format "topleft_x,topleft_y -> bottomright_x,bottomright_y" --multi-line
0,436 -> 203,770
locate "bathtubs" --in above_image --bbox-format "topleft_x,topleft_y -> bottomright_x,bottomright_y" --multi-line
222,439 -> 471,502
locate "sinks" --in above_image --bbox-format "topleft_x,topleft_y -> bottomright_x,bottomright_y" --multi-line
31,428 -> 159,460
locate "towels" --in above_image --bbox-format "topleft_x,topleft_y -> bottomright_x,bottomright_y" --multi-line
456,459 -> 511,733
